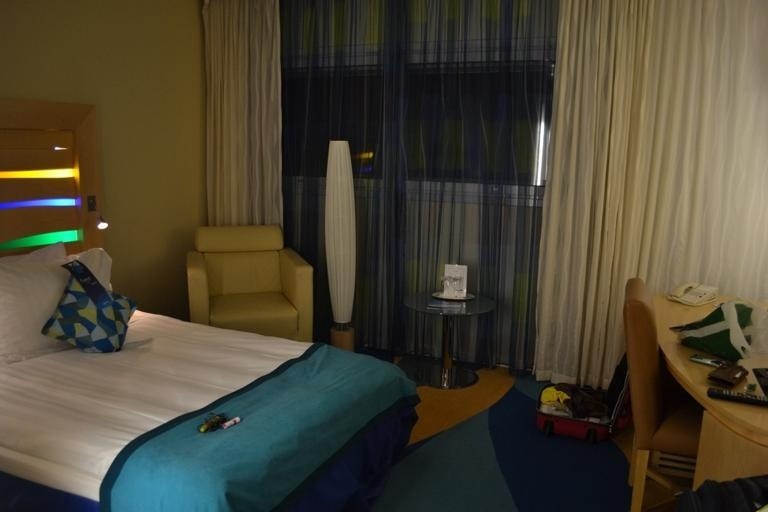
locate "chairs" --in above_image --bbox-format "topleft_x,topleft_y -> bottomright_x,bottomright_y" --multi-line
623,278 -> 702,512
187,224 -> 313,344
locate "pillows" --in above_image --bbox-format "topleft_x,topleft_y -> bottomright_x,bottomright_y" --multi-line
0,241 -> 139,357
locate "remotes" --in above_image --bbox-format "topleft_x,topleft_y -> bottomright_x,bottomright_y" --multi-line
708,387 -> 768,406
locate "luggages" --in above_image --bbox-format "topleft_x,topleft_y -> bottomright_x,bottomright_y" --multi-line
537,352 -> 630,444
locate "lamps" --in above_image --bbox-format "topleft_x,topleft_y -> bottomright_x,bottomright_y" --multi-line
93,212 -> 109,232
320,139 -> 356,352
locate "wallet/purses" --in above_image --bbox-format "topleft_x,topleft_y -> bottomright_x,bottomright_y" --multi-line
708,363 -> 748,387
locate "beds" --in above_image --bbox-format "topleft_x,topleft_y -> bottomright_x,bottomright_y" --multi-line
0,240 -> 422,512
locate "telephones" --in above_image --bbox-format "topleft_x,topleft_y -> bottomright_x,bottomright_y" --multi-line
667,282 -> 718,306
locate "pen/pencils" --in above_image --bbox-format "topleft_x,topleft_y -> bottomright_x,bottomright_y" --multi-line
200,414 -> 241,433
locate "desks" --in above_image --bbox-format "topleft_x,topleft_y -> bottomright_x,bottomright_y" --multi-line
403,293 -> 496,391
653,290 -> 768,512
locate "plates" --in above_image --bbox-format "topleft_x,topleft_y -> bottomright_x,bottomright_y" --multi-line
432,292 -> 476,300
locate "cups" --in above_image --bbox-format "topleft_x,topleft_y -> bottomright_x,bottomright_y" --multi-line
440,275 -> 465,300
